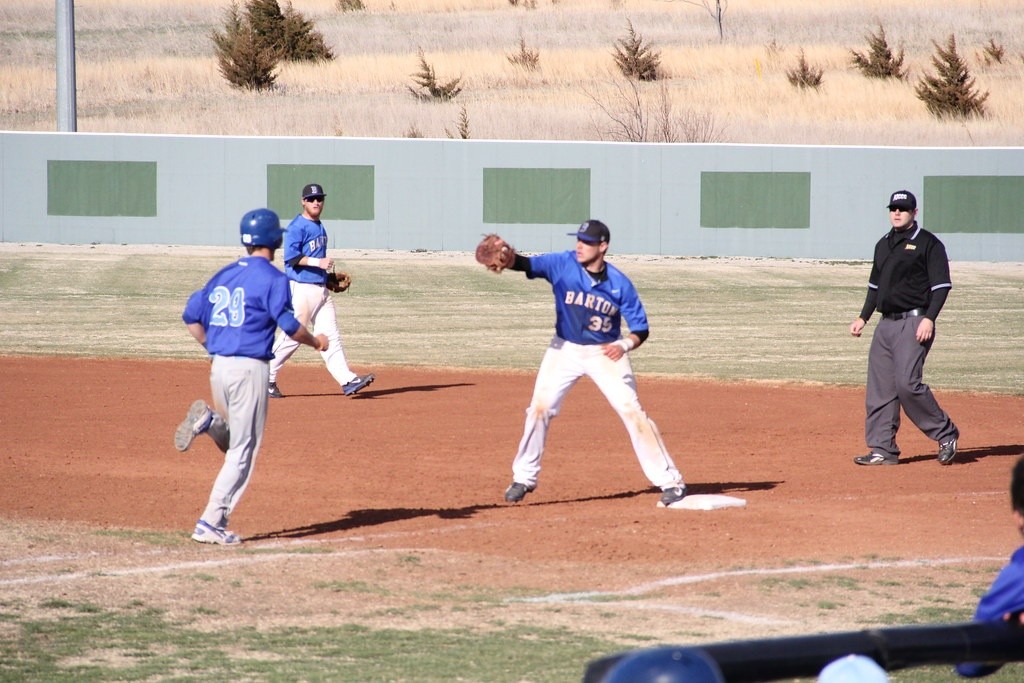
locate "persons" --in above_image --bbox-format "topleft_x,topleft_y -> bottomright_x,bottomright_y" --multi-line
475,219 -> 686,508
817,655 -> 889,682
602,645 -> 724,682
850,190 -> 960,466
268,183 -> 375,398
174,208 -> 328,546
957,455 -> 1024,677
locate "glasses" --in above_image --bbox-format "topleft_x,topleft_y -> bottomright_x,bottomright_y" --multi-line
306,196 -> 323,202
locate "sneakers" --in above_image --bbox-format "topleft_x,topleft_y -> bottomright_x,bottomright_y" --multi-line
854,451 -> 900,465
657,486 -> 688,509
505,483 -> 531,502
191,518 -> 241,545
267,382 -> 282,398
342,372 -> 375,396
175,400 -> 212,452
938,429 -> 960,464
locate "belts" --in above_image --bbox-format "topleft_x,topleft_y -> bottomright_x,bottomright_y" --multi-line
882,309 -> 926,321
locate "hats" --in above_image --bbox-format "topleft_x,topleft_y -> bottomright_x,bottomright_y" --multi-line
886,190 -> 916,209
302,183 -> 327,199
818,654 -> 889,683
566,220 -> 610,244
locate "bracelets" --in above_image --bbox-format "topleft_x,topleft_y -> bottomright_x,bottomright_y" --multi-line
615,338 -> 635,352
308,257 -> 320,267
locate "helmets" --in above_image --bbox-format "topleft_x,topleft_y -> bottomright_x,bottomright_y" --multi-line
239,209 -> 288,250
605,645 -> 725,682
